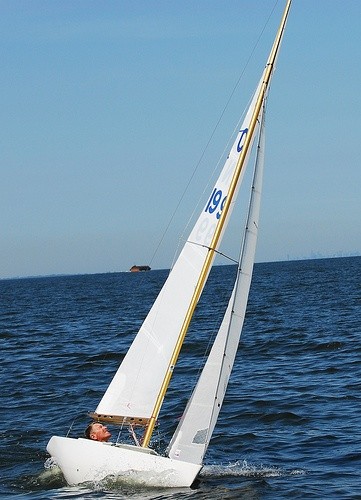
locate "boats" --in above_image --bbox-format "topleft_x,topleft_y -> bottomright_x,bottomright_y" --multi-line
130,265 -> 152,272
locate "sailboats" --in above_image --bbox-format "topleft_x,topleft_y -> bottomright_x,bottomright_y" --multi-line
45,1 -> 293,487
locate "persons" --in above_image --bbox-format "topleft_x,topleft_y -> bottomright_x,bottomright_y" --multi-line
85,422 -> 113,443
